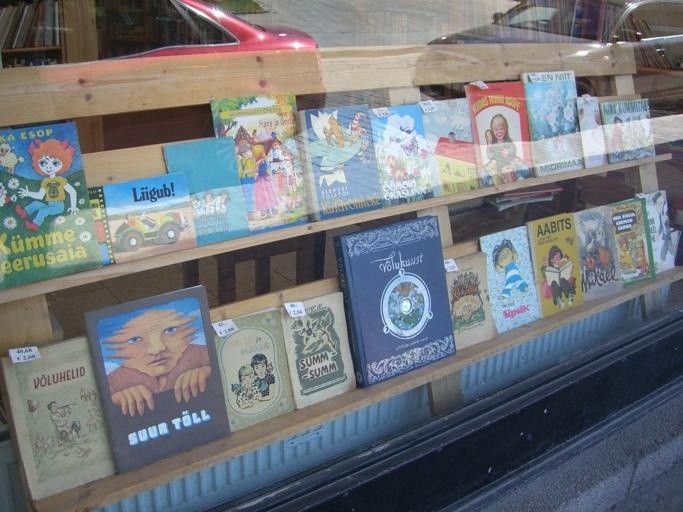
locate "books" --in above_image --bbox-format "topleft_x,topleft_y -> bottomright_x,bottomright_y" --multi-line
3,284 -> 358,500
297,70 -> 654,223
333,215 -> 456,390
443,190 -> 677,352
1,93 -> 312,289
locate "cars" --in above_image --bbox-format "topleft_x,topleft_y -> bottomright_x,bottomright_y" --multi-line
413,1 -> 683,144
0,0 -> 326,153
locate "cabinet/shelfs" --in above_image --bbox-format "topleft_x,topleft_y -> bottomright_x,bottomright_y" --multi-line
1,1 -> 106,154
2,43 -> 682,512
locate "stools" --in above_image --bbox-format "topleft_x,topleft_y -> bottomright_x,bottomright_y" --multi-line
180,231 -> 328,305
